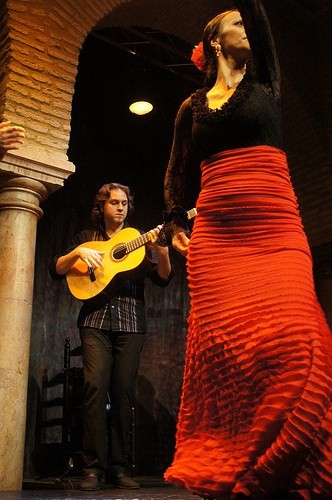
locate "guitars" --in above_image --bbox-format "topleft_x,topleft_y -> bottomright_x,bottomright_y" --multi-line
65,208 -> 199,305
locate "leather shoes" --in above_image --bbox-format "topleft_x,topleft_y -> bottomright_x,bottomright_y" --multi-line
80,468 -> 105,490
105,471 -> 140,489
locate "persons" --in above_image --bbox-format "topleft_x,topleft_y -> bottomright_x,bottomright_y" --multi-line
154,0 -> 332,500
48,183 -> 174,492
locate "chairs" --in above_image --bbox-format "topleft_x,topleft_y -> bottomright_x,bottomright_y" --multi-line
42,337 -> 136,482
